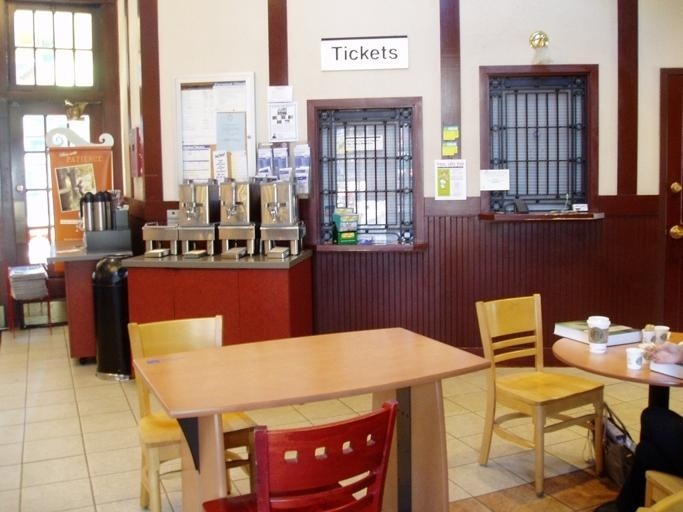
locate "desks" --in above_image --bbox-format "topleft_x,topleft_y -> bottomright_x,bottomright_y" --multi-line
121,249 -> 313,378
48,241 -> 134,358
132,326 -> 491,512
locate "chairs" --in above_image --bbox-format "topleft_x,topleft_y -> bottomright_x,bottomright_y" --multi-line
3,260 -> 52,338
128,315 -> 259,512
476,293 -> 604,497
637,470 -> 683,512
203,401 -> 398,511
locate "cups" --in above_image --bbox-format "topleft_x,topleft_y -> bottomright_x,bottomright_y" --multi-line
655,325 -> 670,345
642,328 -> 656,344
626,348 -> 643,370
587,315 -> 611,353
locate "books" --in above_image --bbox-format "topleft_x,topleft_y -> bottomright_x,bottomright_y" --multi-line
8,263 -> 49,301
648,360 -> 683,381
553,320 -> 641,347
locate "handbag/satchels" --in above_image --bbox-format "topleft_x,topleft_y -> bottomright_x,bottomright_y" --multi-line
589,415 -> 638,490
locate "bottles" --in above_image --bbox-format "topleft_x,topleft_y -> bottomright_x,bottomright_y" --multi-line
104,191 -> 113,230
94,191 -> 107,231
79,192 -> 94,231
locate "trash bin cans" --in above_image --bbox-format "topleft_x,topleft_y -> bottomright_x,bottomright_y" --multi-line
91,254 -> 131,381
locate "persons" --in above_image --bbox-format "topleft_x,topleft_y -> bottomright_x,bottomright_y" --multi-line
77,178 -> 84,196
593,340 -> 683,509
